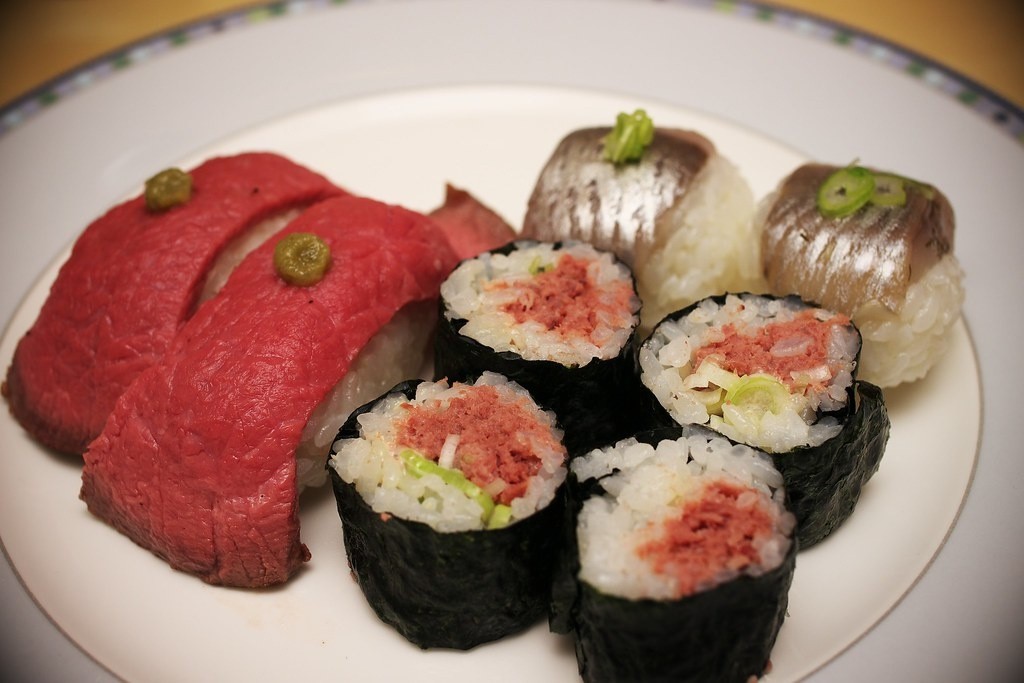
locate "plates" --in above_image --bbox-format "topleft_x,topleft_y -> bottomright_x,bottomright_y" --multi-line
0,0 -> 1024,683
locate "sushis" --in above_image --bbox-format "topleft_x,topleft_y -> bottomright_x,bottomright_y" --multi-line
0,108 -> 969,683
